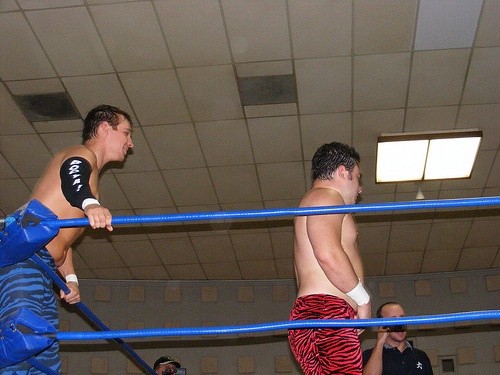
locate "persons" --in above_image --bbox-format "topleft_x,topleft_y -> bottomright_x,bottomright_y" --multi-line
362,301 -> 433,375
287,142 -> 374,375
0,103 -> 134,374
153,357 -> 181,375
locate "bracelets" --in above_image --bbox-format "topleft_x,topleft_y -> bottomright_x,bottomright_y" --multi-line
64,274 -> 79,286
82,197 -> 100,210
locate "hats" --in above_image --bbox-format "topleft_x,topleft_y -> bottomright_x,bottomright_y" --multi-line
153,356 -> 180,369
